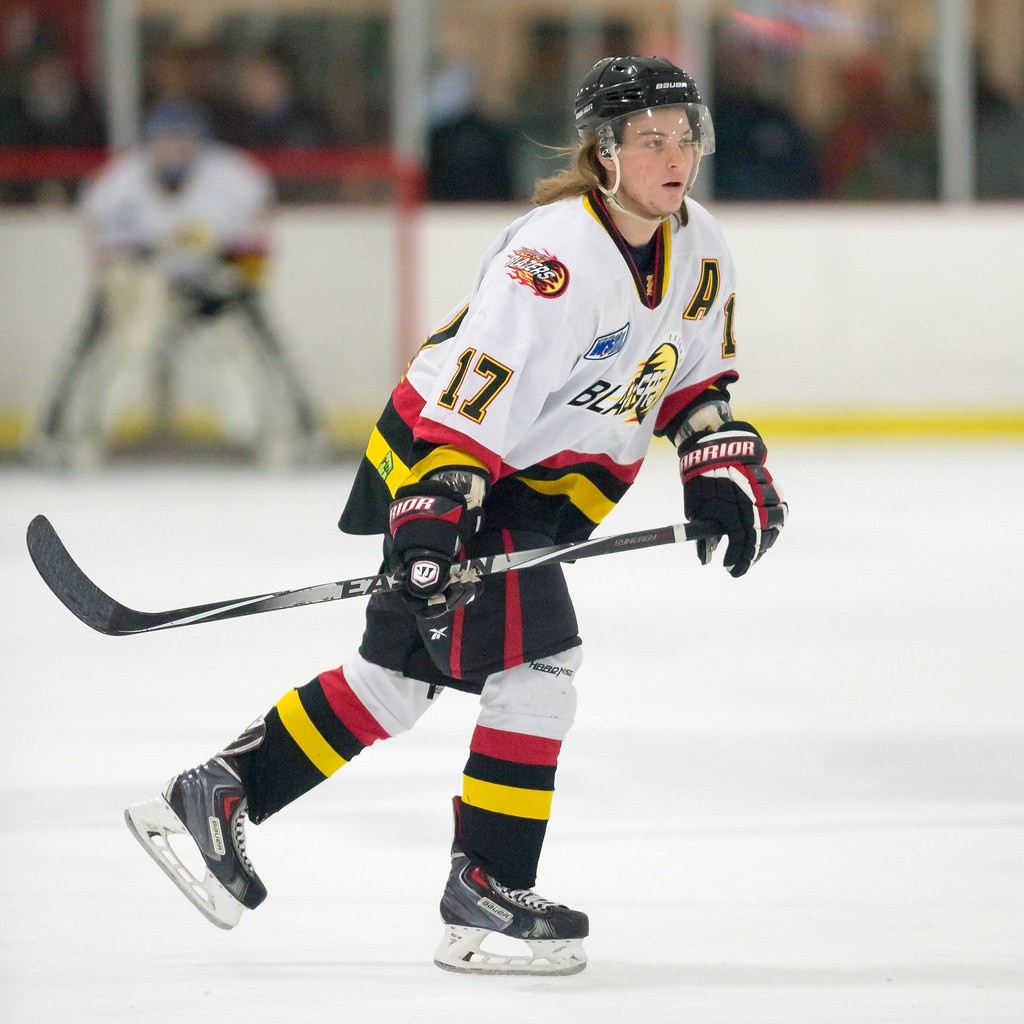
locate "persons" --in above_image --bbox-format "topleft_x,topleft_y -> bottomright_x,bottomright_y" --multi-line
1,14 -> 1024,490
124,54 -> 784,974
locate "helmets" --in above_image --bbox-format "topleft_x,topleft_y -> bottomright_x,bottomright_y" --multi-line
573,54 -> 704,146
140,98 -> 208,137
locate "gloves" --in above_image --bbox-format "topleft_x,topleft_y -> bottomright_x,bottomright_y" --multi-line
383,481 -> 486,620
676,421 -> 790,580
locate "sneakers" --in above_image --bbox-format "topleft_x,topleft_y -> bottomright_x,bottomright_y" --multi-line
124,713 -> 268,931
434,796 -> 590,978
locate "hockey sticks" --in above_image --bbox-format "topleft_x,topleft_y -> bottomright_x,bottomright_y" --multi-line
25,499 -> 790,638
